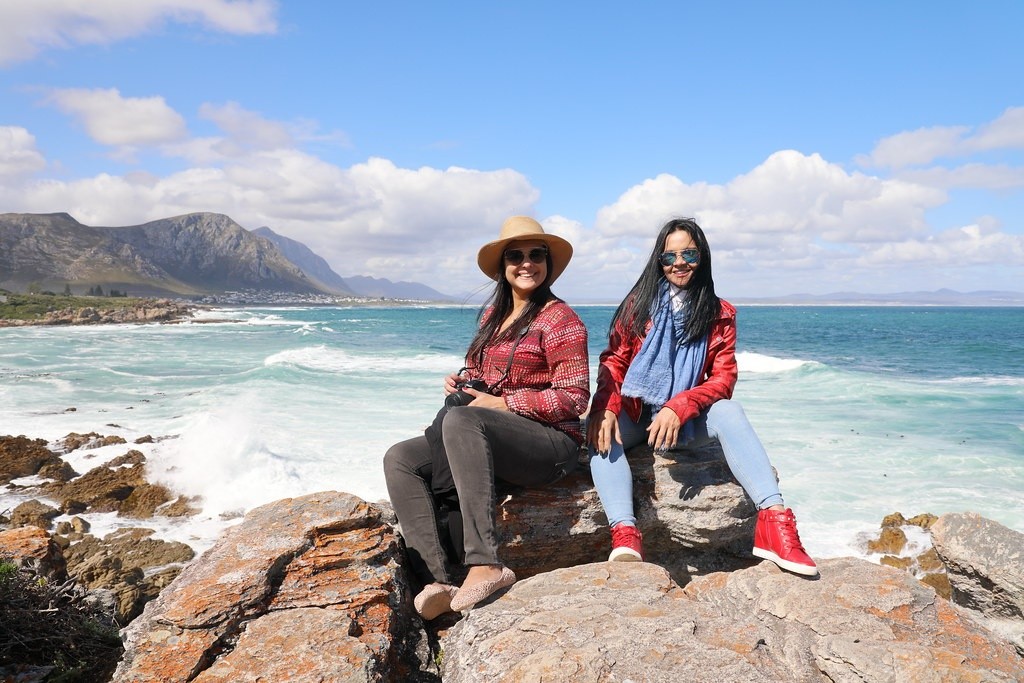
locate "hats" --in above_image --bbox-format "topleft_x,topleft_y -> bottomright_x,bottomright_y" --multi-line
477,216 -> 573,286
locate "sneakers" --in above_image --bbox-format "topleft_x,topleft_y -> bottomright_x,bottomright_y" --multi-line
752,507 -> 817,576
608,525 -> 646,562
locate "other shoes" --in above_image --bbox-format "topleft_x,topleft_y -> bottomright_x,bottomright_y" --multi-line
450,565 -> 516,612
413,584 -> 459,620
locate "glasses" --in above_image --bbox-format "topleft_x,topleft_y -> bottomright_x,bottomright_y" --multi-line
659,249 -> 701,266
504,247 -> 549,263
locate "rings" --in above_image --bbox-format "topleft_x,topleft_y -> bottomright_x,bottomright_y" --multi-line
593,423 -> 596,426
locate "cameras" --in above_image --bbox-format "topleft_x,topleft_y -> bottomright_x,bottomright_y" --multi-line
445,379 -> 493,412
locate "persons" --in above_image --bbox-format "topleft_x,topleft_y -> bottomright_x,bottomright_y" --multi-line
383,216 -> 591,620
586,216 -> 818,576
